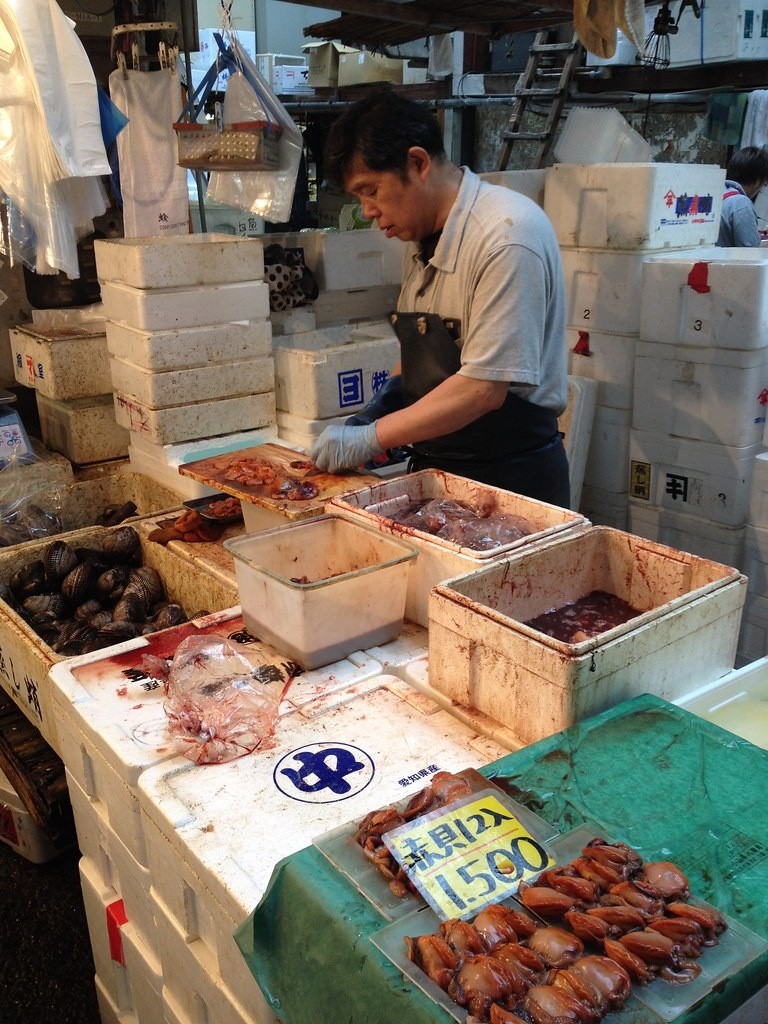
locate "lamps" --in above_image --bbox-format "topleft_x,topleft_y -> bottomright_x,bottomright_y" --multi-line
636,0 -> 679,67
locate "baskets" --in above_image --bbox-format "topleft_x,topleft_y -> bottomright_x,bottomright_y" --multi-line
171,121 -> 283,172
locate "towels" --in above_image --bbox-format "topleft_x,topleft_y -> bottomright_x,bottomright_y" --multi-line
108,68 -> 189,239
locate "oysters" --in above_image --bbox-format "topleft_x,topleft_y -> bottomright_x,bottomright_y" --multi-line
1,526 -> 211,655
355,769 -> 731,1024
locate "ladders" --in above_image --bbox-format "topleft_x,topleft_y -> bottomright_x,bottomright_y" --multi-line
492,31 -> 584,172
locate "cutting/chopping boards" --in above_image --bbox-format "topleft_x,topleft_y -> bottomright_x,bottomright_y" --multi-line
177,442 -> 385,522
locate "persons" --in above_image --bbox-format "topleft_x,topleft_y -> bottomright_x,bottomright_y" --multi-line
715,144 -> 768,247
309,92 -> 572,512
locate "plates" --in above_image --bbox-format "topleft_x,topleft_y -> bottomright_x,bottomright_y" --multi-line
181,493 -> 243,527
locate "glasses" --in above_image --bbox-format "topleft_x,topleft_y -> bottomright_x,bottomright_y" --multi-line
761,183 -> 768,190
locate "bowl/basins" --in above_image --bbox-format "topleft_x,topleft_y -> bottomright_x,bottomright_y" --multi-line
223,512 -> 420,671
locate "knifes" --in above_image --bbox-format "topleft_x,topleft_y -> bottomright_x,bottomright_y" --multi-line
303,467 -> 324,477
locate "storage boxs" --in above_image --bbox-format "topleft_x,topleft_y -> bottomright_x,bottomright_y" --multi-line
198,27 -> 256,91
0,162 -> 768,1024
255,53 -> 309,94
191,69 -> 207,103
338,51 -> 402,86
586,0 -> 768,67
402,59 -> 428,85
300,42 -> 359,88
453,75 -> 487,96
